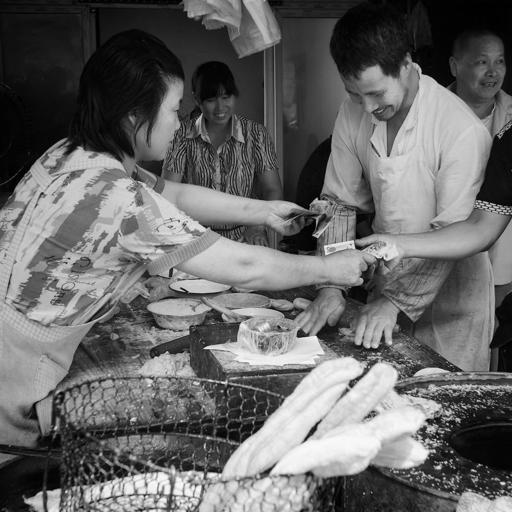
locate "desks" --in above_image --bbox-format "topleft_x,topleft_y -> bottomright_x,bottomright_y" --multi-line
34,285 -> 473,445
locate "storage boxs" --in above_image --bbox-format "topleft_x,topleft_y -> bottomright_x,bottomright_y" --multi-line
189,322 -> 354,418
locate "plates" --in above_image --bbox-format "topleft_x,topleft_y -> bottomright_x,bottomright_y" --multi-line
207,293 -> 269,320
168,277 -> 230,300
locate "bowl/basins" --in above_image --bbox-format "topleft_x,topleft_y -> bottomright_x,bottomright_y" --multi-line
145,298 -> 208,331
238,315 -> 301,356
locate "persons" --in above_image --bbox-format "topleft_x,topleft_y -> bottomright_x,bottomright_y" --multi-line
438,18 -> 511,369
0,27 -> 377,468
286,6 -> 494,374
351,117 -> 511,260
158,58 -> 285,253
288,131 -> 375,302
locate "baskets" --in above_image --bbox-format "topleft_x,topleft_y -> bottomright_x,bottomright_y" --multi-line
53,375 -> 341,512
